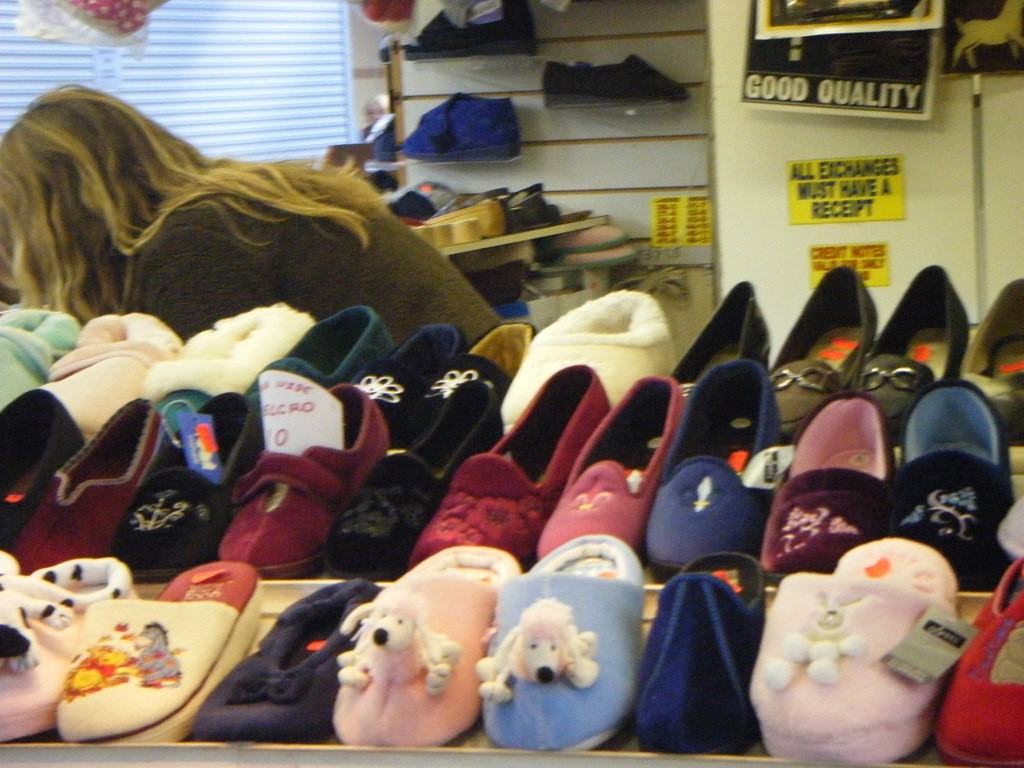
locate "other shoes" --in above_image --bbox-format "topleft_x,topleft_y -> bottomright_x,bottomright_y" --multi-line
359,1 -> 693,260
1,262 -> 1023,767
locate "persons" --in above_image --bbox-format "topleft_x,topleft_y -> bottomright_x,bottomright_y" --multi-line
0,85 -> 503,354
